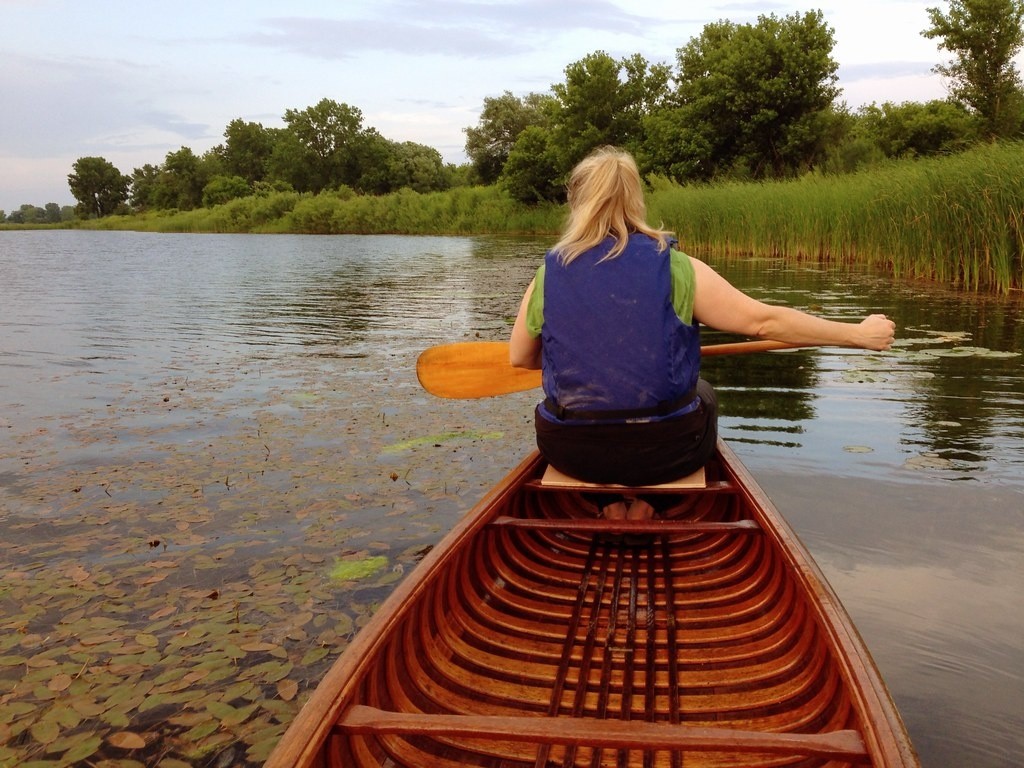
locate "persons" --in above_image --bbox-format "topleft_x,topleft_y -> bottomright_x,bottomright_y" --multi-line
509,148 -> 896,535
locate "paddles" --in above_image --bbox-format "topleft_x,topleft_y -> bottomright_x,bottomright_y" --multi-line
415,340 -> 809,400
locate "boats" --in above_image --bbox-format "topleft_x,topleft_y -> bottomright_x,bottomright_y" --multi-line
262,433 -> 923,768
